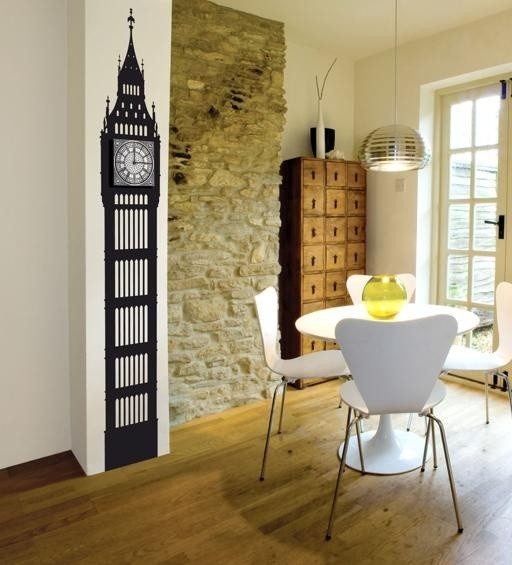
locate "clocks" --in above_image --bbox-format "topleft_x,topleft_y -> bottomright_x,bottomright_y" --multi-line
114,140 -> 155,186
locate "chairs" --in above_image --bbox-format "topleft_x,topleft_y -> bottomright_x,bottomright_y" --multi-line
339,273 -> 428,436
325,315 -> 463,542
407,281 -> 512,432
252,286 -> 366,482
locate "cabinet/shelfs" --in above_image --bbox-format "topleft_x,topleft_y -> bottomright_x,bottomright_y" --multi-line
279,156 -> 367,390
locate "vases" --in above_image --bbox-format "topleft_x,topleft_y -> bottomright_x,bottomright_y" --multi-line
315,99 -> 325,159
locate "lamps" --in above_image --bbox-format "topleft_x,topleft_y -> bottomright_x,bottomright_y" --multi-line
357,0 -> 431,171
